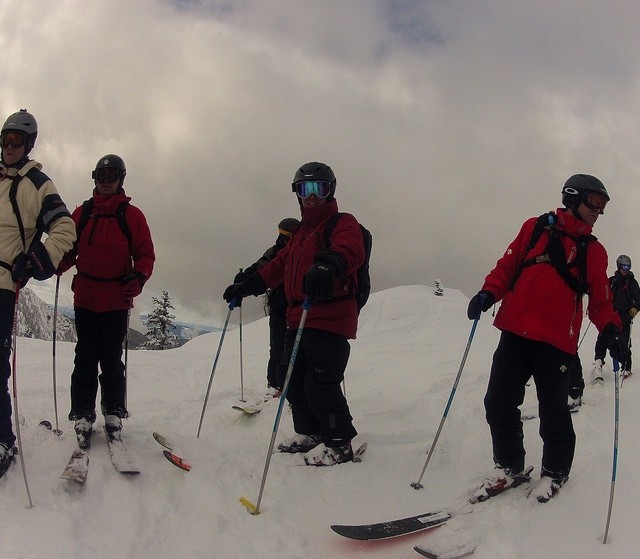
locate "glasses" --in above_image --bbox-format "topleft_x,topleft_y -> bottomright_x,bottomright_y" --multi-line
581,190 -> 607,213
295,181 -> 331,200
0,131 -> 25,148
95,167 -> 120,183
618,263 -> 631,270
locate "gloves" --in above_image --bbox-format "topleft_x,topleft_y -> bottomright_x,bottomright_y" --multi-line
302,261 -> 338,300
122,273 -> 143,302
11,251 -> 33,289
223,266 -> 259,310
602,324 -> 627,359
467,290 -> 494,320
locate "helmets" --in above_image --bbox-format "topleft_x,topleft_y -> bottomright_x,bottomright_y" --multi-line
562,174 -> 610,211
93,154 -> 126,181
617,255 -> 631,268
292,162 -> 336,202
1,109 -> 37,153
277,219 -> 299,243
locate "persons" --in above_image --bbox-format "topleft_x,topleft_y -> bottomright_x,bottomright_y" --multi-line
592,255 -> 640,381
233,218 -> 300,400
55,154 -> 155,448
0,108 -> 78,480
467,173 -> 627,503
222,161 -> 373,466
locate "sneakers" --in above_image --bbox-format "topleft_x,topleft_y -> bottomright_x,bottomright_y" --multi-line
621,371 -> 631,377
74,416 -> 92,439
263,387 -> 281,400
304,442 -> 354,465
568,397 -> 582,412
594,359 -> 604,381
0,442 -> 15,464
479,465 -> 523,494
277,433 -> 322,452
104,412 -> 121,432
537,475 -> 568,502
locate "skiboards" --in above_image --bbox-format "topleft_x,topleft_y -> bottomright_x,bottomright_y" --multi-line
152,431 -> 369,472
58,435 -> 141,482
329,466 -> 569,559
232,390 -> 276,414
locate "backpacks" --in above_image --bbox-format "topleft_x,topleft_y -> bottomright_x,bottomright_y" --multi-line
357,223 -> 372,309
